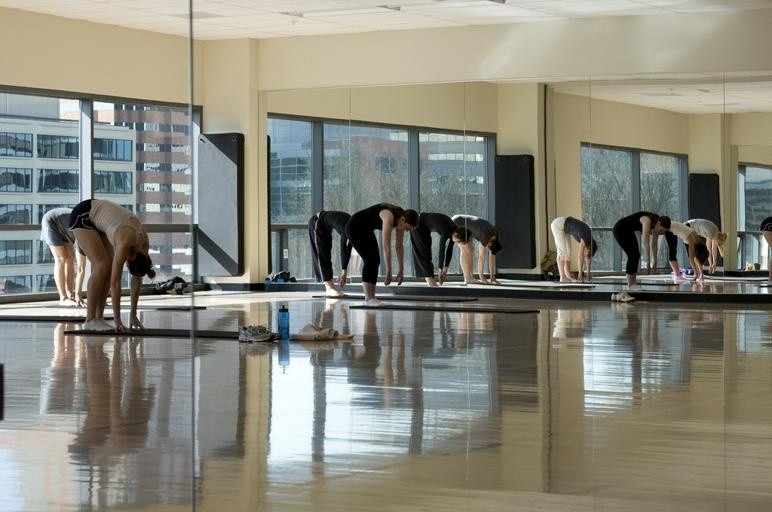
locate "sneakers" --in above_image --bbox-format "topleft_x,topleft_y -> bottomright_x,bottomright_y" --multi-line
238,325 -> 280,342
611,291 -> 636,302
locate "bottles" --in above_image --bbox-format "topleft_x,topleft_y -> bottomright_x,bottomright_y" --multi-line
265,278 -> 270,292
277,275 -> 284,282
277,305 -> 289,343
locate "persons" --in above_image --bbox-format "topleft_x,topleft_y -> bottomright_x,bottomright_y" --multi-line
549,309 -> 661,360
344,203 -> 420,307
663,220 -> 709,284
37,316 -> 156,471
37,207 -> 109,308
306,208 -> 355,297
613,211 -> 672,287
409,211 -> 473,287
66,198 -> 156,335
309,300 -> 494,409
551,215 -> 598,285
682,219 -> 728,279
450,214 -> 502,285
759,216 -> 772,287
